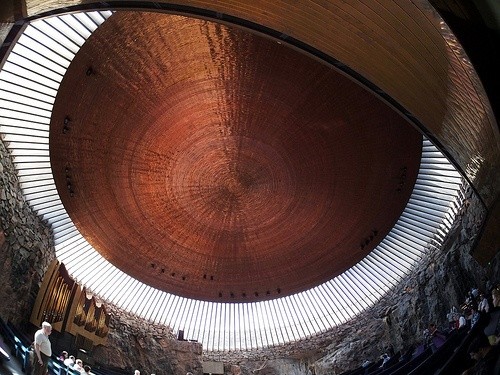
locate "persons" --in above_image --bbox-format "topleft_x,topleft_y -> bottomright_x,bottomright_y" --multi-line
31,322 -> 52,375
23,342 -> 34,375
60,351 -> 92,375
362,345 -> 396,368
423,275 -> 500,375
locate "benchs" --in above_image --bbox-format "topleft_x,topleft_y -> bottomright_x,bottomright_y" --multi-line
0,315 -> 135,375
345,293 -> 500,375
